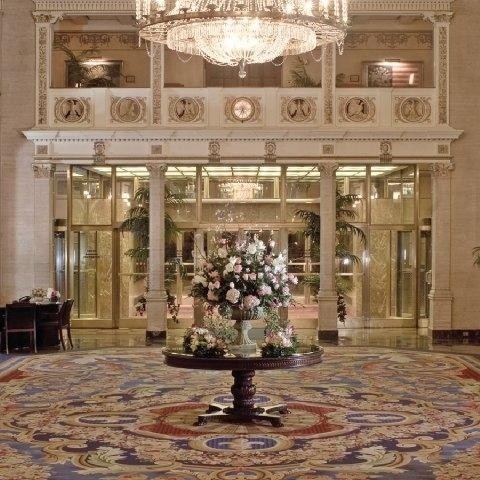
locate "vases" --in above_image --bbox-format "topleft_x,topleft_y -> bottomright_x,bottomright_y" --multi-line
49,296 -> 58,303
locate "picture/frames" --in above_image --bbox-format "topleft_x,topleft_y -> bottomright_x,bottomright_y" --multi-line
360,58 -> 427,88
63,58 -> 125,89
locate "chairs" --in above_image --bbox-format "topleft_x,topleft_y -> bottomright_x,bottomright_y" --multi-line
4,297 -> 75,355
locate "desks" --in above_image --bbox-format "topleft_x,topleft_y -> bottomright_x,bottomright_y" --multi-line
0,302 -> 62,351
161,342 -> 326,428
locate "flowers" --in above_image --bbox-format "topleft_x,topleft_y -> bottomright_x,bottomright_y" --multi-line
29,287 -> 61,305
182,223 -> 304,356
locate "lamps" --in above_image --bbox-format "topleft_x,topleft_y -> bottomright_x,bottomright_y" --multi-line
134,0 -> 352,80
